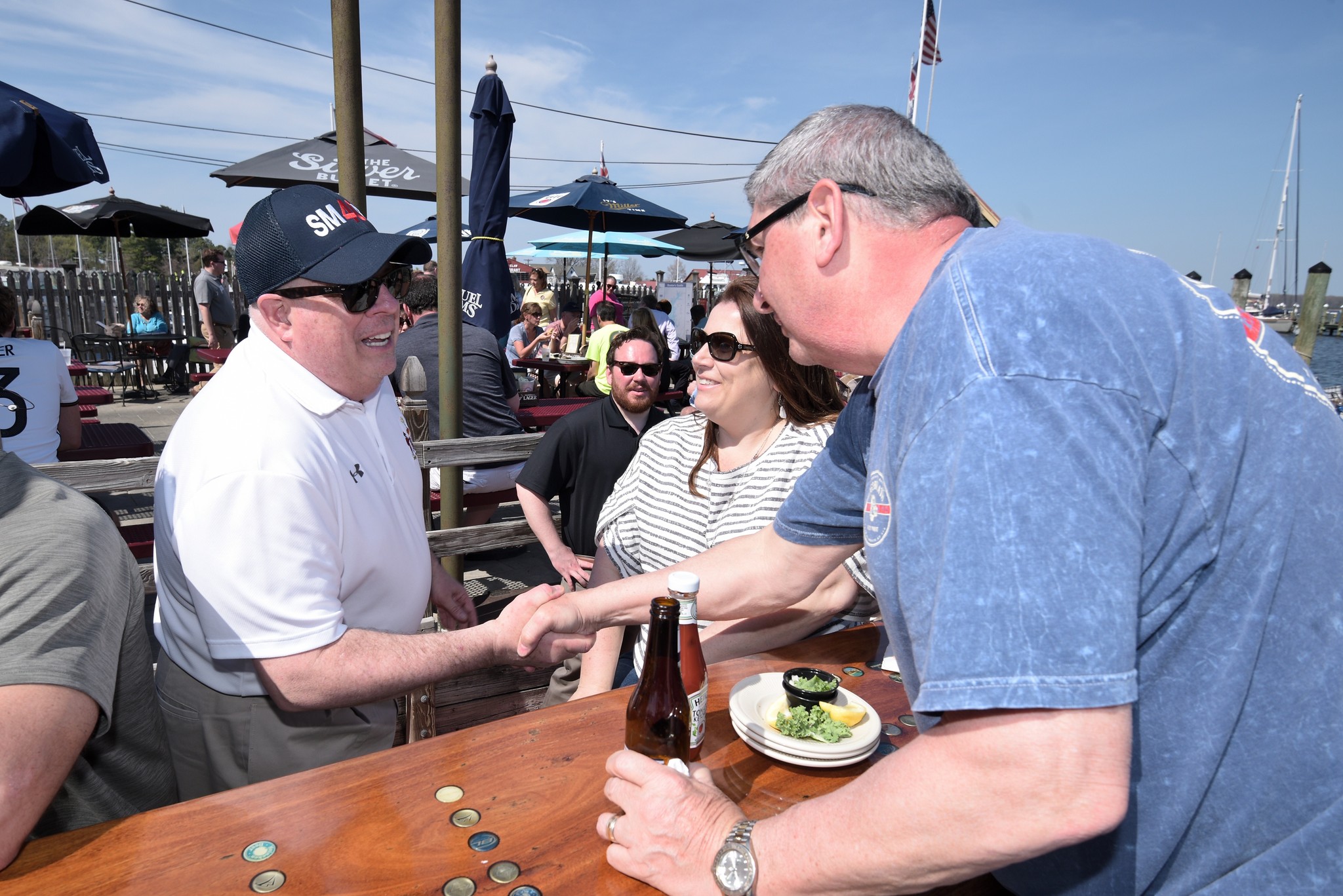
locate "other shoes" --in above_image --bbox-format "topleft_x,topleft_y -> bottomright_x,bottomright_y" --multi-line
152,376 -> 171,384
670,400 -> 679,406
168,385 -> 188,395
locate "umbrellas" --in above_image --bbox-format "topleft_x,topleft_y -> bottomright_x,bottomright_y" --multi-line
395,214 -> 473,243
507,167 -> 756,347
14,186 -> 214,387
0,81 -> 110,198
209,127 -> 470,202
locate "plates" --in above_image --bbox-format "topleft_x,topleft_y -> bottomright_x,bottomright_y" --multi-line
728,672 -> 882,768
556,356 -> 590,365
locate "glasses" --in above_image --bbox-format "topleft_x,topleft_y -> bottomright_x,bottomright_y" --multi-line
273,263 -> 413,312
734,183 -> 876,280
690,326 -> 757,361
136,303 -> 146,307
529,312 -> 542,317
532,269 -> 540,274
606,284 -> 617,289
209,260 -> 225,265
613,360 -> 662,377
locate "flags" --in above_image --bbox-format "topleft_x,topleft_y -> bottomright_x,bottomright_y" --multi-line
14,197 -> 31,213
600,146 -> 609,179
907,0 -> 941,120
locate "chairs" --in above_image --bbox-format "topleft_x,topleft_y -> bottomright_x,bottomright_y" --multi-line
43,325 -> 211,406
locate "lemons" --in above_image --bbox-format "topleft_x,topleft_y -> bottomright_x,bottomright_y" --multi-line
768,693 -> 792,731
818,701 -> 866,727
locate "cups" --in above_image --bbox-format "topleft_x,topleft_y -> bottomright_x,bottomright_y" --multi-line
59,348 -> 71,366
542,348 -> 550,362
518,376 -> 535,392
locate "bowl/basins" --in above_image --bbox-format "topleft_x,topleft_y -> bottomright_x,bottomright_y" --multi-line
782,680 -> 838,716
783,666 -> 840,701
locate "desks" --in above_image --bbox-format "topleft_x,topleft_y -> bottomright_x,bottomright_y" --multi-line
517,397 -> 602,428
95,333 -> 188,398
73,385 -> 113,405
196,348 -> 233,363
67,359 -> 88,377
512,358 -> 590,397
57,423 -> 156,462
0,623 -> 919,895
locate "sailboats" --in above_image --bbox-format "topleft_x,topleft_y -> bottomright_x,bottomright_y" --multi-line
1242,93 -> 1304,331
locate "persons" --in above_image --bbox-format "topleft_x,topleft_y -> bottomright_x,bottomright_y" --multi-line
189,249 -> 236,397
0,450 -> 180,871
0,284 -> 121,527
390,259 -> 879,710
126,295 -> 170,390
152,183 -> 597,804
516,106 -> 1343,896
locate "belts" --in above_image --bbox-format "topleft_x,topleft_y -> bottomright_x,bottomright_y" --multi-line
213,322 -> 232,328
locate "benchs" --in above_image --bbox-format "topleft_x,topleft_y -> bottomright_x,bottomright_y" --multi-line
190,374 -> 214,382
430,489 -> 519,510
116,523 -> 154,560
79,405 -> 101,425
653,390 -> 685,418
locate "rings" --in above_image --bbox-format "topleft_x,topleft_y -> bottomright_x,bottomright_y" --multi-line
606,813 -> 623,843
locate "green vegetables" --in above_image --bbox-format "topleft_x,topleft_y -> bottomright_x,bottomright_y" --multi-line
793,674 -> 837,692
776,705 -> 852,743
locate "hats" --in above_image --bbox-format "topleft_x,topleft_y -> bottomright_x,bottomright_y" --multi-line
562,301 -> 583,312
235,184 -> 432,305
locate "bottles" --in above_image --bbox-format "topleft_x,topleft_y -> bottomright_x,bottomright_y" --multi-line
667,570 -> 709,762
623,596 -> 692,770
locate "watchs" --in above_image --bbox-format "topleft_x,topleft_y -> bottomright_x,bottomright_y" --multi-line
711,819 -> 755,896
